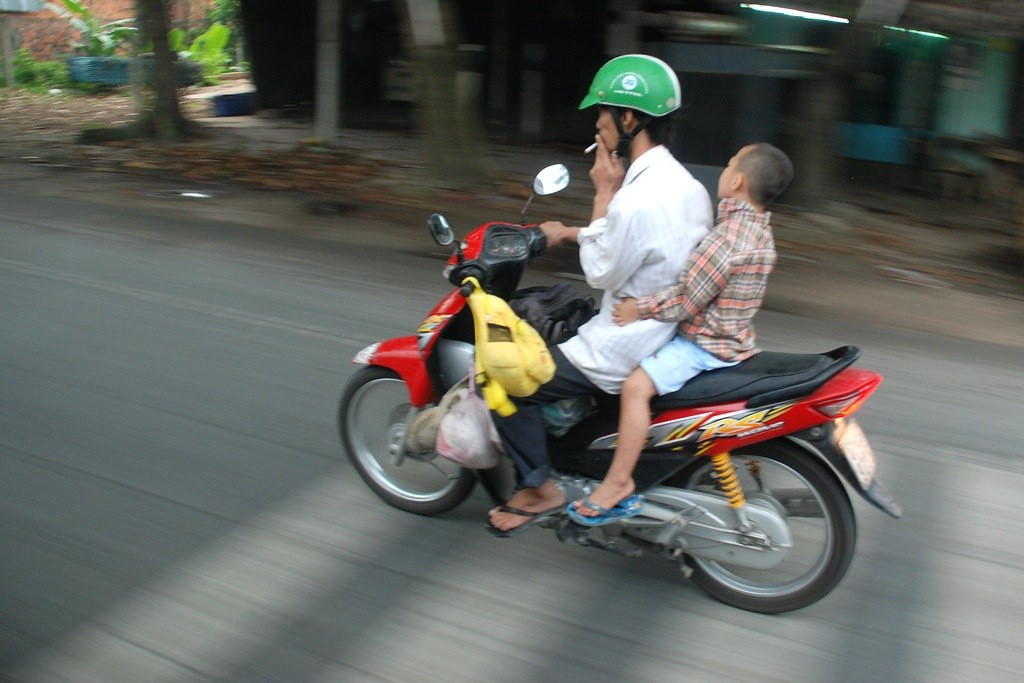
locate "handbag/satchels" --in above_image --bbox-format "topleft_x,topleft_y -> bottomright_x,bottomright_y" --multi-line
435,392 -> 500,468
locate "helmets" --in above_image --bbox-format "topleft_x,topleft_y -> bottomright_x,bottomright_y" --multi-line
576,55 -> 683,120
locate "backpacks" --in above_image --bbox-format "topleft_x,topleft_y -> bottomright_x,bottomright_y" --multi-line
460,277 -> 556,417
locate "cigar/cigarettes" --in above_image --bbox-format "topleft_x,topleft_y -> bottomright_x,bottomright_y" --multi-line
582,141 -> 598,153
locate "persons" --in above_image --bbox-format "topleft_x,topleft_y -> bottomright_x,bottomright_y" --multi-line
564,140 -> 796,529
484,54 -> 714,540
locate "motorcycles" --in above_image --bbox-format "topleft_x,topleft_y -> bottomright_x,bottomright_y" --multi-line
336,165 -> 905,616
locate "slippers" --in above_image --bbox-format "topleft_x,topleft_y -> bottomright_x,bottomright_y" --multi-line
485,502 -> 566,537
569,495 -> 645,525
